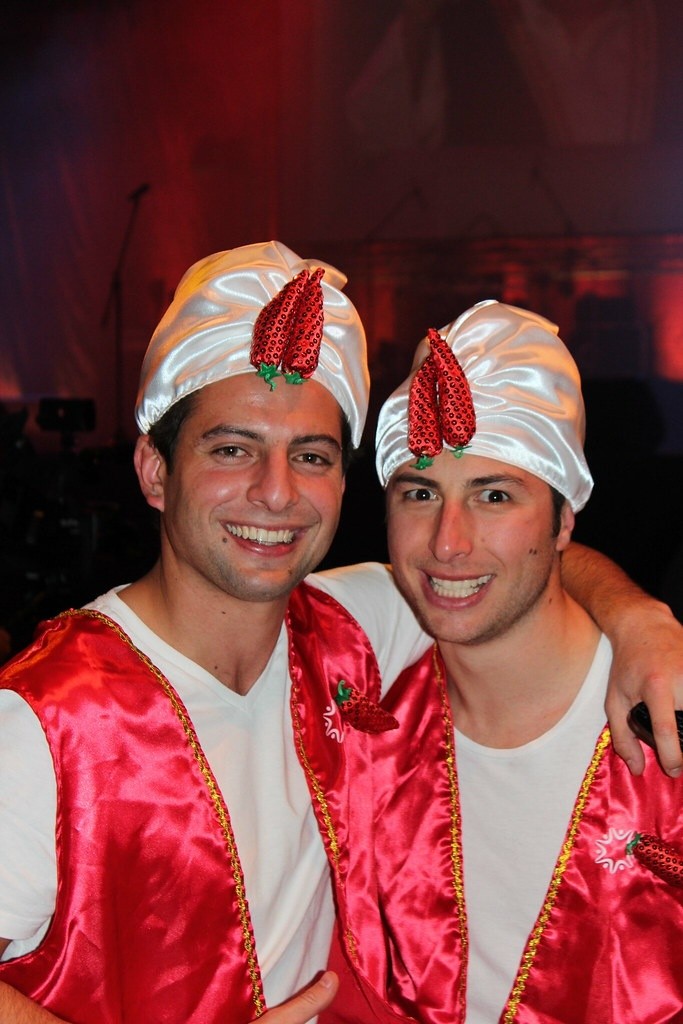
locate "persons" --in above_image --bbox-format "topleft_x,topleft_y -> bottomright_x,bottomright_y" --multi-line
375,300 -> 683,1024
0,239 -> 683,1024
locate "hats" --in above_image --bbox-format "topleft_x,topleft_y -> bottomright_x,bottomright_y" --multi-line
374,298 -> 594,513
134,240 -> 370,450
